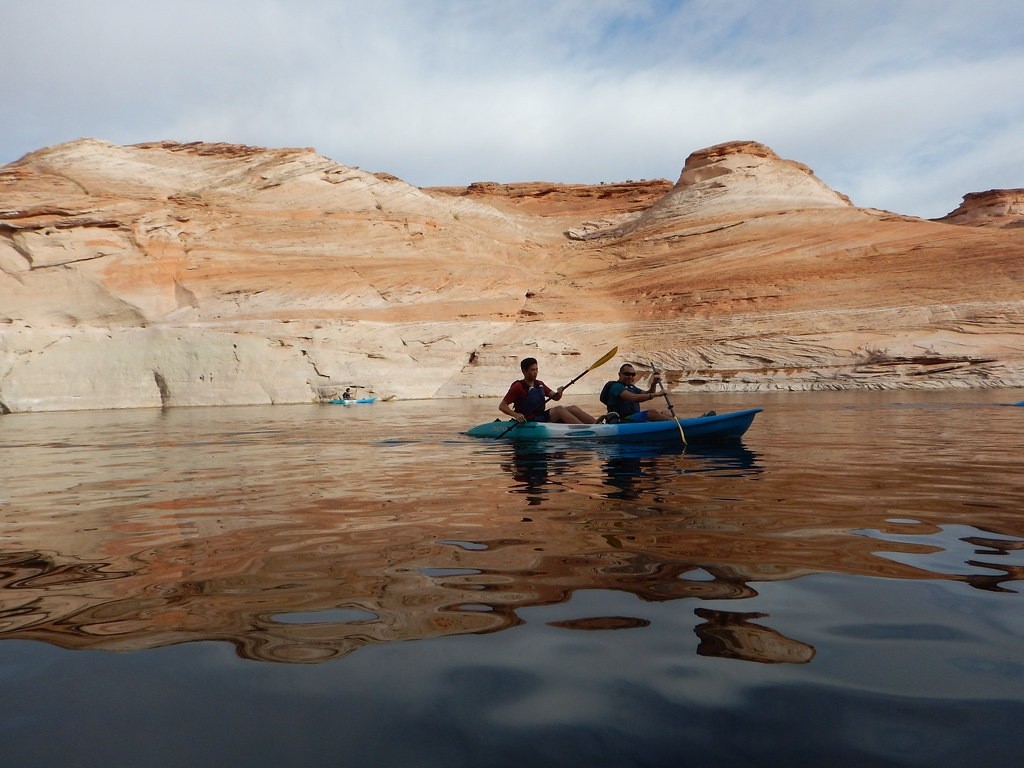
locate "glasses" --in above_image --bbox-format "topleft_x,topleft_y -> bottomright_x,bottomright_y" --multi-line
621,371 -> 636,377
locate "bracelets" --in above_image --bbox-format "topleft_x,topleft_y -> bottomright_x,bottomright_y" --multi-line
649,394 -> 652,400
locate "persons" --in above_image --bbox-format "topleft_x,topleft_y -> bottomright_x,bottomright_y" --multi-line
343,388 -> 356,400
607,364 -> 672,421
499,358 -> 621,424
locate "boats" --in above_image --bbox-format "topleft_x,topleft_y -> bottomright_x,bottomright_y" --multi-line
466,407 -> 764,440
330,397 -> 377,404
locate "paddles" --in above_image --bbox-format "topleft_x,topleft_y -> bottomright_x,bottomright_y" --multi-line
494,346 -> 618,441
649,360 -> 688,445
